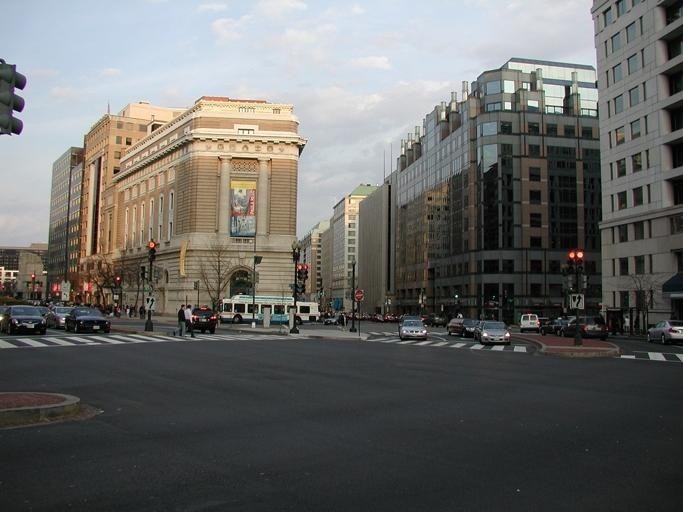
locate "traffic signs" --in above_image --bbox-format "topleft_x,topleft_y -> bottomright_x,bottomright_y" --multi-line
454,293 -> 459,304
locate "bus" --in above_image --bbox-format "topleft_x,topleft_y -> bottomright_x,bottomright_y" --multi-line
216,294 -> 320,325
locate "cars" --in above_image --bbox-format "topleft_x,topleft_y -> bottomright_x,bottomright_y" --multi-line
186,306 -> 217,333
320,311 -> 607,345
646,319 -> 683,344
0,299 -> 112,334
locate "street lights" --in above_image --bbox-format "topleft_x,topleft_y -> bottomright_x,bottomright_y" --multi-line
290,239 -> 302,333
349,260 -> 356,331
251,253 -> 262,324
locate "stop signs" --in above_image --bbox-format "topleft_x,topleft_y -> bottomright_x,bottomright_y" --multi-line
354,290 -> 364,302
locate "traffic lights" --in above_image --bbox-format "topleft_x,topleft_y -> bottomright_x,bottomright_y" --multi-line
297,264 -> 303,281
303,264 -> 307,280
291,284 -> 299,299
31,274 -> 34,285
193,280 -> 198,290
299,282 -> 305,295
567,249 -> 575,274
576,249 -> 584,273
148,242 -> 155,262
115,276 -> 120,286
139,267 -> 144,280
0,61 -> 27,137
508,295 -> 513,309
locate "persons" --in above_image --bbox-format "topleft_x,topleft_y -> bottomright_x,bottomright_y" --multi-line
320,311 -> 409,324
624,316 -> 630,336
446,310 -> 452,332
172,304 -> 186,337
72,300 -> 145,319
456,312 -> 463,318
183,304 -> 195,337
